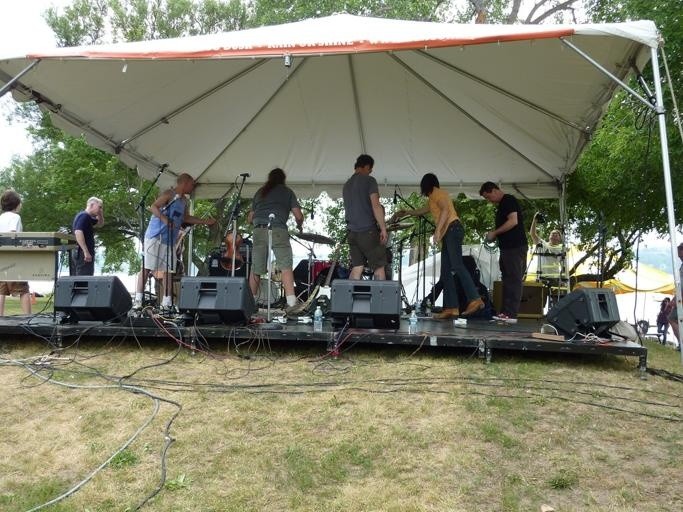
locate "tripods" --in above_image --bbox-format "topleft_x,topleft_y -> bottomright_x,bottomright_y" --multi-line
523,242 -> 542,283
397,194 -> 436,319
296,243 -> 320,306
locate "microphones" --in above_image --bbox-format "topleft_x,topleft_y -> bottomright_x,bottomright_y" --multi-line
160,193 -> 180,212
268,213 -> 275,228
159,162 -> 170,168
394,190 -> 397,204
311,204 -> 314,219
240,172 -> 251,177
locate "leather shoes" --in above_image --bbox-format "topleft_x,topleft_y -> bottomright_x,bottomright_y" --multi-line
434,307 -> 458,320
461,298 -> 485,317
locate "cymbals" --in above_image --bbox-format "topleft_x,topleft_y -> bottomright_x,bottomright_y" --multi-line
385,222 -> 414,231
295,232 -> 336,244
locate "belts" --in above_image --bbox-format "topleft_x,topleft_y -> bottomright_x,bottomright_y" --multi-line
254,224 -> 266,228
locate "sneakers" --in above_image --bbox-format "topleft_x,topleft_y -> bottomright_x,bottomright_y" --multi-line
159,305 -> 178,314
491,311 -> 508,320
497,314 -> 517,323
131,299 -> 142,311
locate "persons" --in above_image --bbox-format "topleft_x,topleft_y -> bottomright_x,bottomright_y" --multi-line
247,168 -> 305,308
342,153 -> 388,281
72,196 -> 104,275
377,204 -> 403,280
656,298 -> 671,344
396,173 -> 486,319
530,212 -> 563,307
131,174 -> 217,310
0,190 -> 32,315
667,243 -> 683,351
479,182 -> 527,324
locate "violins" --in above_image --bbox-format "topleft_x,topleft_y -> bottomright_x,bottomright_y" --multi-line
219,199 -> 244,271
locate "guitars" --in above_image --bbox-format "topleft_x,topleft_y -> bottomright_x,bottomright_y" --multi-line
310,230 -> 350,316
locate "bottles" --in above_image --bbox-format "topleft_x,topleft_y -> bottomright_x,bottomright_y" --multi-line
424,298 -> 431,317
313,306 -> 323,333
409,310 -> 418,335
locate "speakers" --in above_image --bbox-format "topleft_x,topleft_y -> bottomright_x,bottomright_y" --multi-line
180,277 -> 255,324
331,279 -> 401,329
55,275 -> 132,321
547,288 -> 620,336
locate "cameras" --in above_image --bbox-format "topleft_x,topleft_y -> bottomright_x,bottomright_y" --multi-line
537,212 -> 546,224
